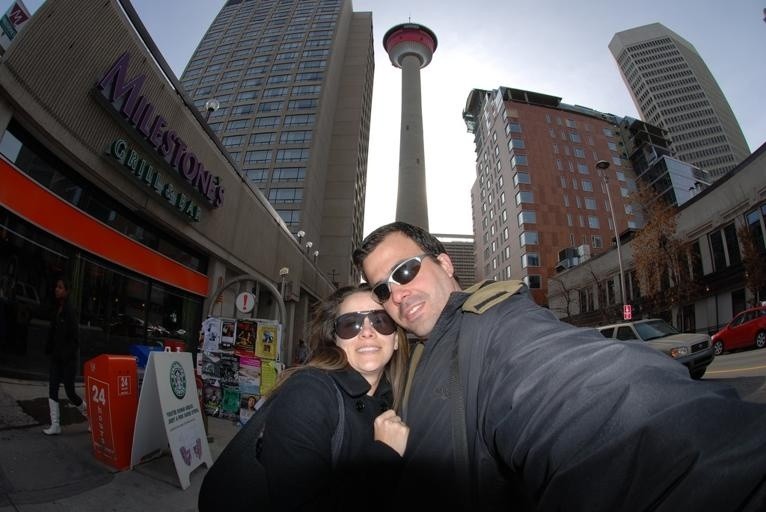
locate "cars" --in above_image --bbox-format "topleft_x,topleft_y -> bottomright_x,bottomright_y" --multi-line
0,277 -> 171,353
711,305 -> 766,356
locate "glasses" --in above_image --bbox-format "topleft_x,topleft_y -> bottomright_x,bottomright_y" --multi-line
370,252 -> 431,308
333,309 -> 400,340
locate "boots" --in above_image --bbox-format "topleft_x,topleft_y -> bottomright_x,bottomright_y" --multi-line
74,400 -> 95,432
42,397 -> 62,437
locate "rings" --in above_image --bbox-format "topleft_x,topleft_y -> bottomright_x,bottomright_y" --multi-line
399,420 -> 406,427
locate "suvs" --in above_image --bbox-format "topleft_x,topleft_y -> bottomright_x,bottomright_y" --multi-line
589,318 -> 714,380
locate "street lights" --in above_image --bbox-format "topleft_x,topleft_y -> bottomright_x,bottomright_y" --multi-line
204,98 -> 221,125
276,267 -> 290,328
595,155 -> 630,321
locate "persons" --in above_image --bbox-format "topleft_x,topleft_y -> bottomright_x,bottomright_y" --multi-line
227,324 -> 234,337
191,280 -> 409,512
263,329 -> 273,343
222,324 -> 228,336
207,389 -> 218,404
293,337 -> 313,365
42,277 -> 93,435
351,221 -> 765,509
237,329 -> 255,345
245,395 -> 258,410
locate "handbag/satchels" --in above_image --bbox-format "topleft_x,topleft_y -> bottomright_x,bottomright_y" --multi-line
196,369 -> 346,510
